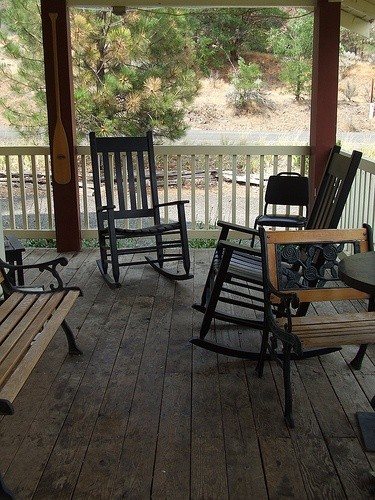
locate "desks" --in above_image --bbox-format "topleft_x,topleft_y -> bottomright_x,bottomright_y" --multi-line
338,250 -> 375,452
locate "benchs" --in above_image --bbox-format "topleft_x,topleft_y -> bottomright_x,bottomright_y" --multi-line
255,223 -> 375,427
0,257 -> 84,416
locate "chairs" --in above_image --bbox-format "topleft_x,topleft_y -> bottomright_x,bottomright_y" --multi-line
89,130 -> 194,289
188,145 -> 363,361
250,172 -> 310,249
